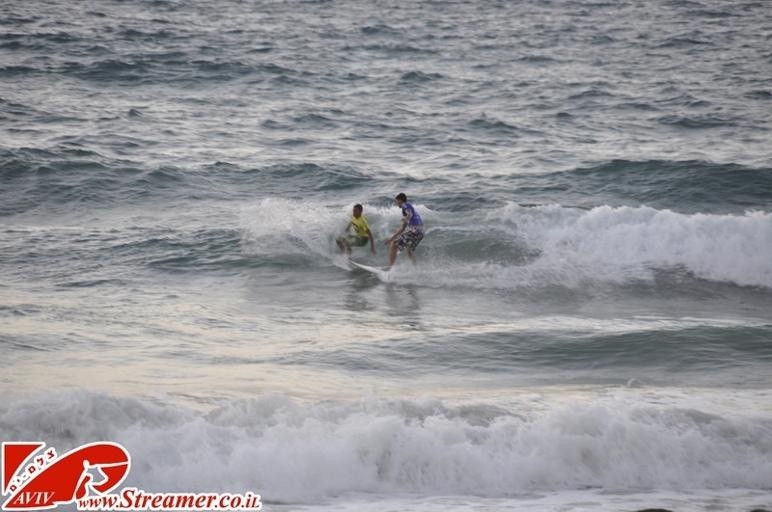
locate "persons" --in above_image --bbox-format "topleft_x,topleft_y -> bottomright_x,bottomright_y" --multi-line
335,203 -> 378,257
381,192 -> 427,272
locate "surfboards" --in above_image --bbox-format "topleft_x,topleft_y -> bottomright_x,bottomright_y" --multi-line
347,257 -> 389,278
